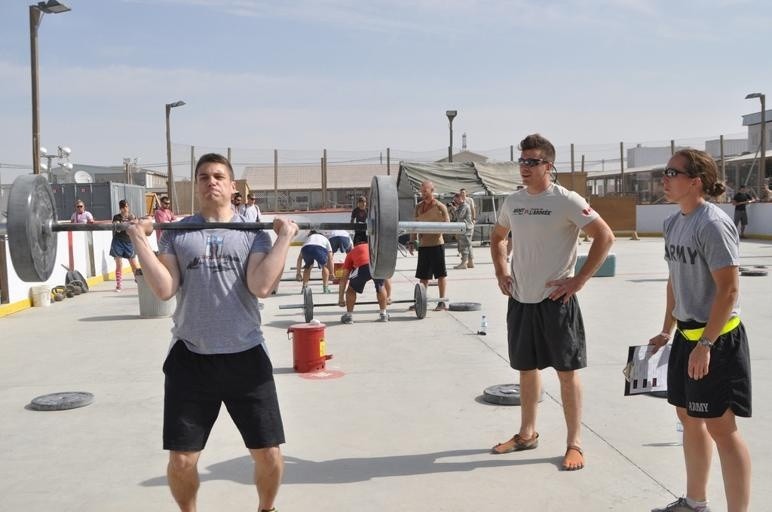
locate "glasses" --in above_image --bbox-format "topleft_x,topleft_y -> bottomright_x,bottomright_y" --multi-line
76,206 -> 83,208
518,157 -> 544,168
249,198 -> 256,200
236,199 -> 242,202
163,201 -> 170,205
664,167 -> 688,178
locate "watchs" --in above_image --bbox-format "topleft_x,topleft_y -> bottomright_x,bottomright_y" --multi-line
697,337 -> 715,349
406,241 -> 414,245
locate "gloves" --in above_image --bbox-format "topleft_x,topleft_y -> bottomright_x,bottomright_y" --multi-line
328,273 -> 336,281
295,273 -> 303,281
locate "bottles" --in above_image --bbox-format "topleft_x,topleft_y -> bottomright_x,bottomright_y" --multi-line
481,315 -> 488,332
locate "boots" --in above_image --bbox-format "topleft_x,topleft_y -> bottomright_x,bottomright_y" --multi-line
468,258 -> 475,268
453,258 -> 468,269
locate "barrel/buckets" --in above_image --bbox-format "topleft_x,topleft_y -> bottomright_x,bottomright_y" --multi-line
333,263 -> 345,283
32,285 -> 51,306
287,323 -> 327,371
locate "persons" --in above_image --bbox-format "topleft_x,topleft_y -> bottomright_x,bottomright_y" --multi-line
732,185 -> 756,239
407,179 -> 450,312
647,149 -> 753,512
155,197 -> 183,245
490,134 -> 615,471
447,188 -> 476,270
109,200 -> 143,292
350,196 -> 368,245
296,230 -> 336,295
327,230 -> 358,280
505,185 -> 524,263
338,241 -> 393,324
759,184 -> 772,202
124,153 -> 300,512
232,192 -> 261,223
70,200 -> 94,224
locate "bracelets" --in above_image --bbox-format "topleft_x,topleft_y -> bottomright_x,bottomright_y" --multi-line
659,332 -> 672,340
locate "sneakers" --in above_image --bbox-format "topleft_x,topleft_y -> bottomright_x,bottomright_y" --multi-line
491,431 -> 540,455
380,312 -> 391,322
650,495 -> 711,512
301,285 -> 308,294
340,313 -> 353,324
323,285 -> 332,294
115,285 -> 122,292
561,441 -> 585,472
433,301 -> 448,311
408,304 -> 416,311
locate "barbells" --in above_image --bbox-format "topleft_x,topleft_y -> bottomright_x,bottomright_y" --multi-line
0,173 -> 465,283
279,282 -> 449,323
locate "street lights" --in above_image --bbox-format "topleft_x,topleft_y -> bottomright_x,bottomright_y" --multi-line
37,142 -> 72,187
165,98 -> 188,215
26,1 -> 74,175
745,92 -> 766,203
444,109 -> 459,165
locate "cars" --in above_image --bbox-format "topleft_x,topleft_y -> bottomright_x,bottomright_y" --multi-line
604,190 -> 668,207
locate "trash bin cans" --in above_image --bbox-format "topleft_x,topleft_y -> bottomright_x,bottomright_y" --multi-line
135,267 -> 177,317
